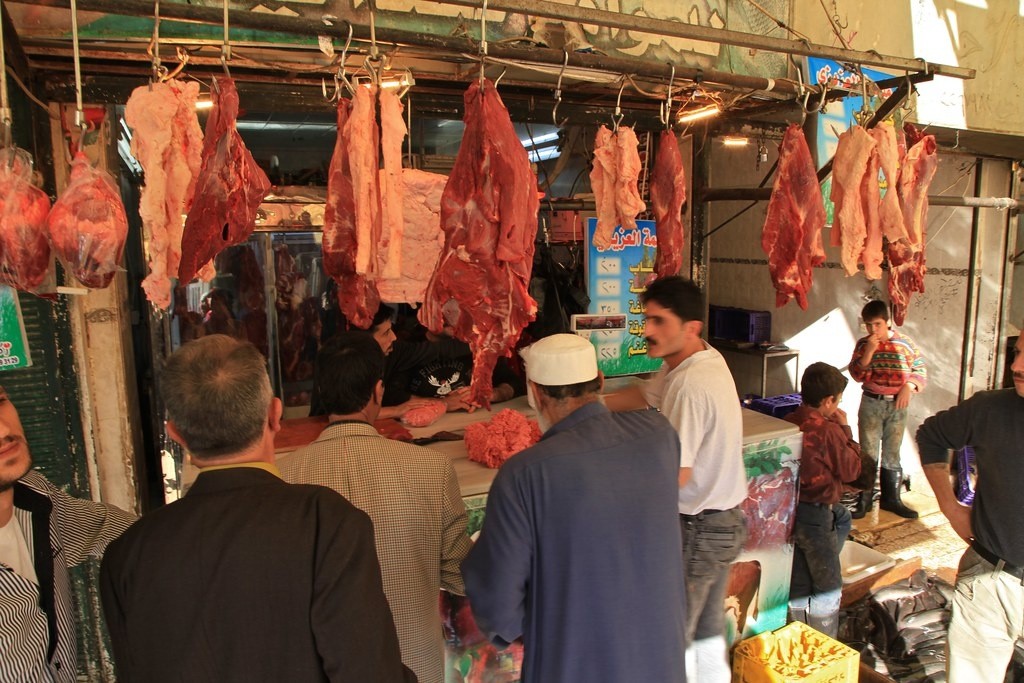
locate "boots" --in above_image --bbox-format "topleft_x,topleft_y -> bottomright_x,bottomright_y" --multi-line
880,467 -> 918,519
786,601 -> 840,640
850,467 -> 878,521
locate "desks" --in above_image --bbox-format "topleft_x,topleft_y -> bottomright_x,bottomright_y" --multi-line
181,387 -> 804,683
707,338 -> 801,397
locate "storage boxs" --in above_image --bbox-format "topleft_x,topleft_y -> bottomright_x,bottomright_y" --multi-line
958,446 -> 975,506
733,621 -> 861,683
779,392 -> 802,407
838,538 -> 896,584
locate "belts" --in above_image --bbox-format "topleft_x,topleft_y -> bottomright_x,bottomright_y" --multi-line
863,390 -> 898,402
971,539 -> 1024,582
704,508 -> 721,516
803,501 -> 833,510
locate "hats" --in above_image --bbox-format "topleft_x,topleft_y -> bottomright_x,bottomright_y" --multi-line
519,333 -> 599,385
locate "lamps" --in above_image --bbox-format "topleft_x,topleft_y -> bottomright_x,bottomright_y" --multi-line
675,86 -> 724,123
351,51 -> 415,88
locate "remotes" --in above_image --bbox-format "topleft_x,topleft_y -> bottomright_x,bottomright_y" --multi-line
759,346 -> 789,351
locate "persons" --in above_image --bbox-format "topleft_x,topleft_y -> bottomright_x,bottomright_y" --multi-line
394,298 -> 526,415
307,301 -> 443,424
595,274 -> 749,674
781,359 -> 864,642
273,329 -> 476,682
915,324 -> 1024,683
97,334 -> 423,683
1,378 -> 147,682
847,299 -> 929,520
457,331 -> 690,682
